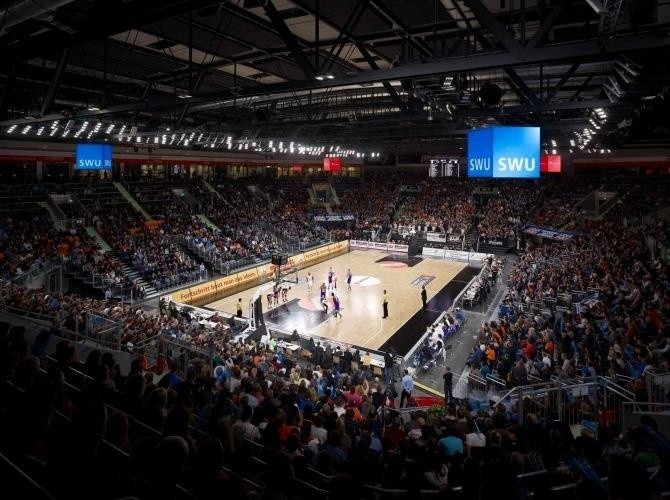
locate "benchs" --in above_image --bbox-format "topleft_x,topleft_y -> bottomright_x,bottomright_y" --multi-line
1,356 -> 586,500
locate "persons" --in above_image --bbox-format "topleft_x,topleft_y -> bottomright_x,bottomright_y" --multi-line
2,167 -> 669,499
